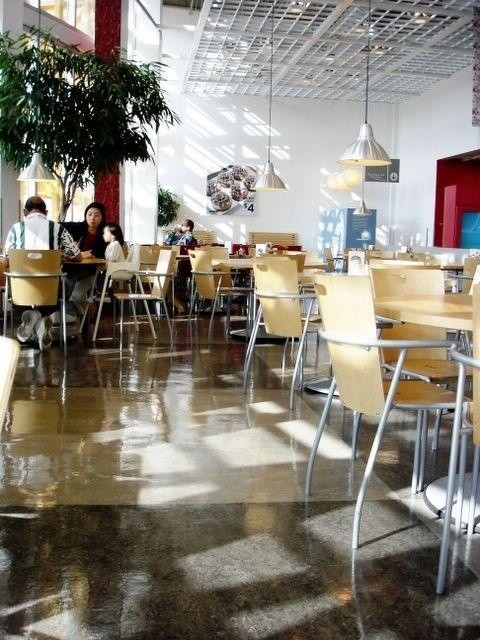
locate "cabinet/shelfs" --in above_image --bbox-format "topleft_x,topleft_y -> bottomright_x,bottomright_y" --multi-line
321,208 -> 376,264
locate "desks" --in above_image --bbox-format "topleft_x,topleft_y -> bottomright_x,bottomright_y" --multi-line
374,296 -> 479,534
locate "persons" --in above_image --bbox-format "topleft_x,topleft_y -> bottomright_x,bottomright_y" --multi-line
94,221 -> 127,301
1,194 -> 83,354
52,201 -> 111,337
164,219 -> 200,314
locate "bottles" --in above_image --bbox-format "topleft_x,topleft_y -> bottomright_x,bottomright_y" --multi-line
239,244 -> 244,259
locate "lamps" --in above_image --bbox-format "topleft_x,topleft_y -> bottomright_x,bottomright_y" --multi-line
16,0 -> 57,182
352,166 -> 372,216
336,0 -> 390,166
249,0 -> 289,191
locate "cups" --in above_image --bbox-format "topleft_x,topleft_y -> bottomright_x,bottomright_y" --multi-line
333,259 -> 346,274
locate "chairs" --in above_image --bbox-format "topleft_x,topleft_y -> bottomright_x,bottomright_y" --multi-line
1,336 -> 20,433
354,268 -> 475,492
436,283 -> 479,592
0,246 -> 252,347
242,246 -> 480,437
304,272 -> 478,550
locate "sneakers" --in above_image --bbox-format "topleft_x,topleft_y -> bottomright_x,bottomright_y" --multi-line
94,291 -> 111,302
81,313 -> 88,337
34,315 -> 53,351
16,309 -> 41,343
